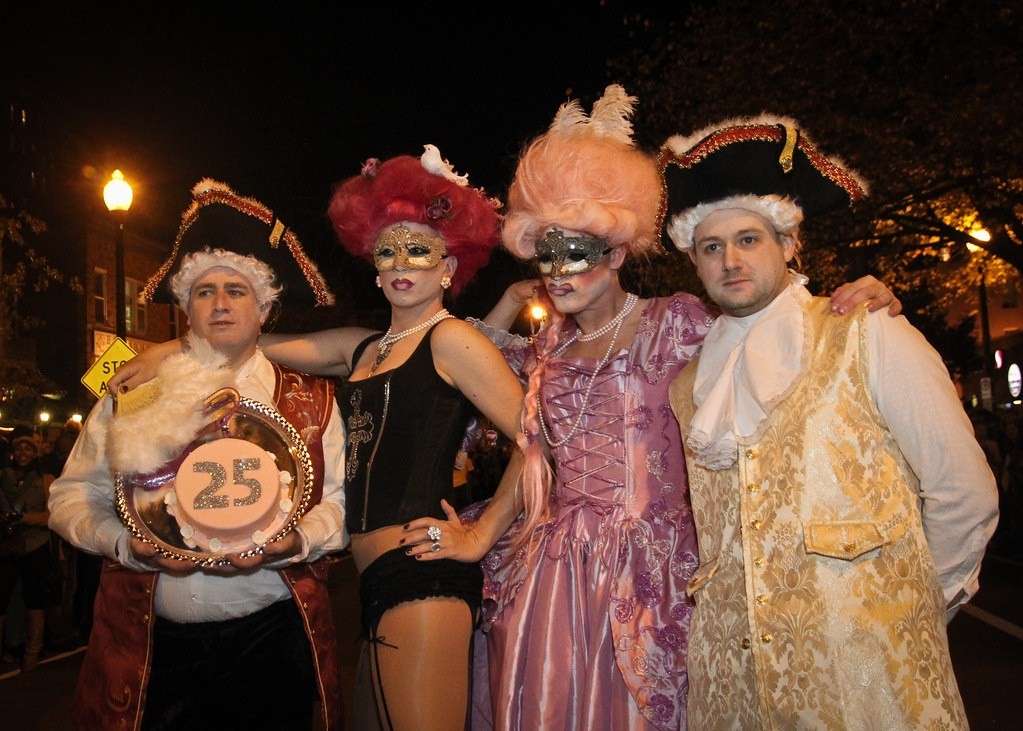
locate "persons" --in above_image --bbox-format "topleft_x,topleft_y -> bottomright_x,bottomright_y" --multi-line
0,421 -> 104,674
101,145 -> 548,731
478,125 -> 902,731
659,107 -> 998,731
44,181 -> 353,731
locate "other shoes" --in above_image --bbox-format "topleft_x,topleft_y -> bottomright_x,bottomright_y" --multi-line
69,630 -> 82,643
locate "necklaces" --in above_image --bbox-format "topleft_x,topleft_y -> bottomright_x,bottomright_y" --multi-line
536,292 -> 638,447
365,308 -> 455,374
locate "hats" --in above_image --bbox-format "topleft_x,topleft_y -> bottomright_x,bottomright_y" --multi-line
9,425 -> 37,449
655,113 -> 867,253
143,179 -> 334,317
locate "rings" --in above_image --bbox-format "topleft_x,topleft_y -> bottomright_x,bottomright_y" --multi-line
431,542 -> 442,552
428,526 -> 442,540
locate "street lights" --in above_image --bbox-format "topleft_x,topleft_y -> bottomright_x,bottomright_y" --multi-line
966,227 -> 1001,416
40,409 -> 51,441
103,170 -> 133,342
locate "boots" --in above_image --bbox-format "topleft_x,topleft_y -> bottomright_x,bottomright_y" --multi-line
0,616 -> 7,652
22,608 -> 46,672
